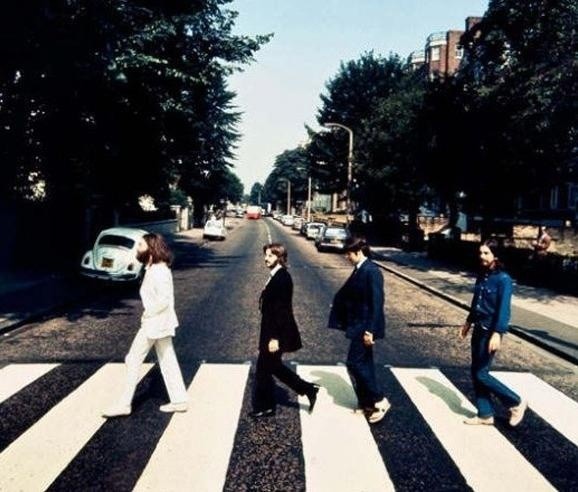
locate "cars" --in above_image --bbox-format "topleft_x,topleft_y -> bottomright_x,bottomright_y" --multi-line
203,219 -> 227,241
227,204 -> 352,252
78,226 -> 151,282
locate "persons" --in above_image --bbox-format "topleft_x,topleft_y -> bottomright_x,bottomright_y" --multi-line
462,239 -> 528,428
102,233 -> 189,417
247,240 -> 320,419
328,238 -> 393,424
532,227 -> 551,255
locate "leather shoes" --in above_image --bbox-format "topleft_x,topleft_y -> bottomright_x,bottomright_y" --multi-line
102,406 -> 133,418
248,406 -> 275,419
307,384 -> 320,414
159,402 -> 187,414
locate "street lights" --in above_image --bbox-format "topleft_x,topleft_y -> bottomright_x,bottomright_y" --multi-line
324,122 -> 353,225
296,167 -> 311,222
279,179 -> 291,216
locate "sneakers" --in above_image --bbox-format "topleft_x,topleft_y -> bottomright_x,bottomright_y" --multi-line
464,416 -> 495,425
354,397 -> 391,423
509,398 -> 528,428
